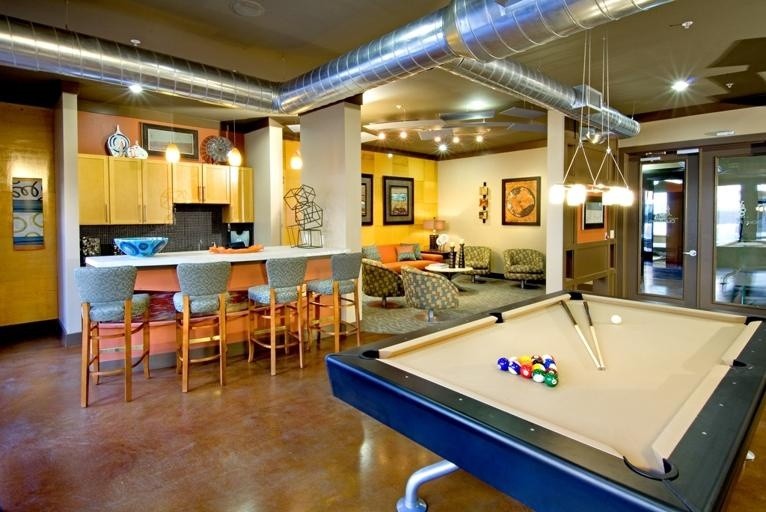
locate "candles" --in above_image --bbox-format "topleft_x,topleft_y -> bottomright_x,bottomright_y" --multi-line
450,241 -> 455,247
459,238 -> 464,244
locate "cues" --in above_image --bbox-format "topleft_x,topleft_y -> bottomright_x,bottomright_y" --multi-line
560,300 -> 600,369
583,302 -> 606,369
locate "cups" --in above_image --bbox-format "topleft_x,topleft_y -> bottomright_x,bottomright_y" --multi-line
429,234 -> 438,250
193,238 -> 218,251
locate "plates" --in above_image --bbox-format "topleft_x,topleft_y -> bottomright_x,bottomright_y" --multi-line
204,136 -> 235,163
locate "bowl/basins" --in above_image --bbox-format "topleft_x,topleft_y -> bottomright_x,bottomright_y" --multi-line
113,237 -> 169,257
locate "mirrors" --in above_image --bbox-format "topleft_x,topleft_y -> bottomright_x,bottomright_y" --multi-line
653,180 -> 683,271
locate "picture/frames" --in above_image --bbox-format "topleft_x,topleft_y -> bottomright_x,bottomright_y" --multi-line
139,120 -> 200,160
382,175 -> 415,225
501,176 -> 541,226
361,173 -> 374,226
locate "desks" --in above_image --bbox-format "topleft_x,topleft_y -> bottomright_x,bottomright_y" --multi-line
421,250 -> 457,265
424,264 -> 473,293
326,287 -> 766,511
82,244 -> 358,373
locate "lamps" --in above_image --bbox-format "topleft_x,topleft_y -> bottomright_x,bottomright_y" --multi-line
422,216 -> 446,250
549,18 -> 633,208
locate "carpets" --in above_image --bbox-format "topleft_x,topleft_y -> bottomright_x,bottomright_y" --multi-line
359,276 -> 546,336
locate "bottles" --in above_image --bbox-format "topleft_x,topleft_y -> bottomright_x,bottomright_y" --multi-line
449,246 -> 456,268
112,242 -> 124,255
458,243 -> 466,268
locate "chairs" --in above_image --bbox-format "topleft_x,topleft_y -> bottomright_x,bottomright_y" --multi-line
308,251 -> 366,358
361,257 -> 407,310
445,246 -> 492,284
502,248 -> 546,290
401,265 -> 461,323
71,265 -> 152,410
172,261 -> 233,395
248,255 -> 310,378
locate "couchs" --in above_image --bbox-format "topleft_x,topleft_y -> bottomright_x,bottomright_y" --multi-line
360,242 -> 443,274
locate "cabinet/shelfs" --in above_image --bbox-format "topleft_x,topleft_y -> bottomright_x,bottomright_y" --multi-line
172,162 -> 231,204
224,166 -> 254,225
77,152 -> 111,226
107,154 -> 174,226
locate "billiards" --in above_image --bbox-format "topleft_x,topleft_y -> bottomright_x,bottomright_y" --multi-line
611,315 -> 621,325
499,354 -> 558,388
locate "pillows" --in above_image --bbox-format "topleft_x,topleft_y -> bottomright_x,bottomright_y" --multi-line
394,245 -> 417,262
400,243 -> 423,260
361,244 -> 383,264
